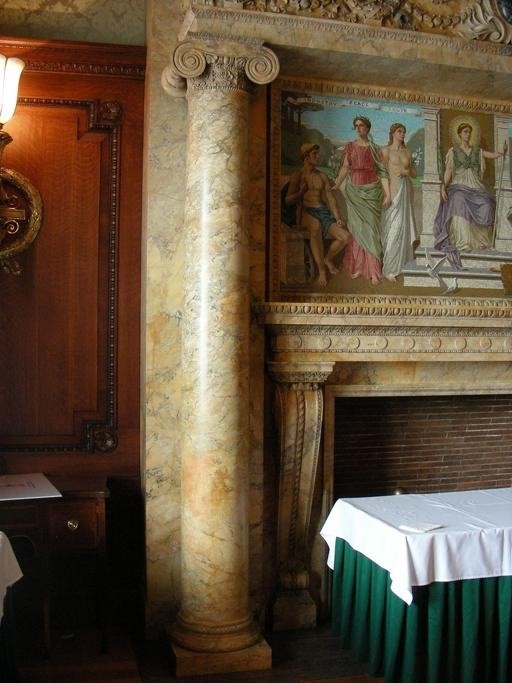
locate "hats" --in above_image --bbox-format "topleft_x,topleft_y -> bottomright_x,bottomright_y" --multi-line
298,141 -> 321,157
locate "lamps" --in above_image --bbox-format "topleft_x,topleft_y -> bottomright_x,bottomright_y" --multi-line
0,51 -> 24,167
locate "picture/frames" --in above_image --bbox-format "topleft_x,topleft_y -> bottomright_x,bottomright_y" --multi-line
267,72 -> 510,317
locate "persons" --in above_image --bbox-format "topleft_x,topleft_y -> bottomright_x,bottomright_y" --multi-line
328,115 -> 391,285
286,143 -> 350,287
381,123 -> 420,283
441,123 -> 508,252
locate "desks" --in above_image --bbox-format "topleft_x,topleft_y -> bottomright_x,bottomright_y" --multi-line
331,487 -> 511,683
0,470 -> 112,658
